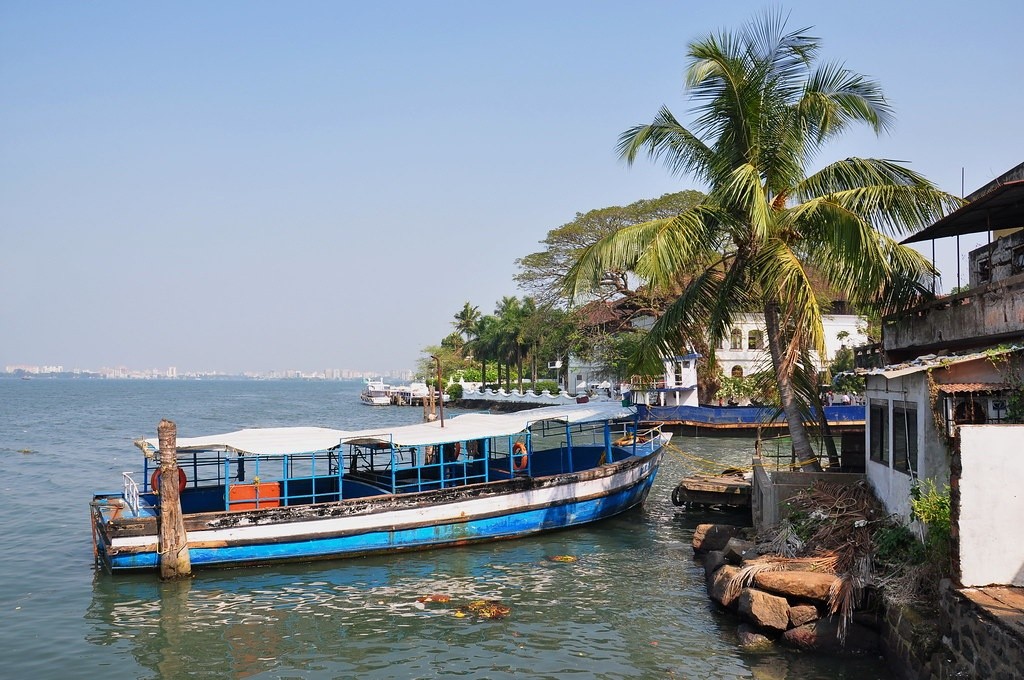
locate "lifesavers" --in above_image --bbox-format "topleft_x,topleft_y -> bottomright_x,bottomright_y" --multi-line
512,442 -> 528,470
671,483 -> 687,508
615,434 -> 640,446
449,442 -> 461,462
150,466 -> 187,495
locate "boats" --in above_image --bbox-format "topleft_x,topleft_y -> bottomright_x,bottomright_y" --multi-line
88,355 -> 673,575
361,378 -> 450,406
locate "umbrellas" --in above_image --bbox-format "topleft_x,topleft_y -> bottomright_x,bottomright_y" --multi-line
576,381 -> 587,391
597,381 -> 610,390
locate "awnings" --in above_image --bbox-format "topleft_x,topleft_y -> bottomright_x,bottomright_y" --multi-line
898,178 -> 1024,301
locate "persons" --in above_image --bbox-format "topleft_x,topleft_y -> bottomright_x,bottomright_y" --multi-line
851,394 -> 860,406
841,392 -> 850,406
828,392 -> 834,406
728,396 -> 734,406
